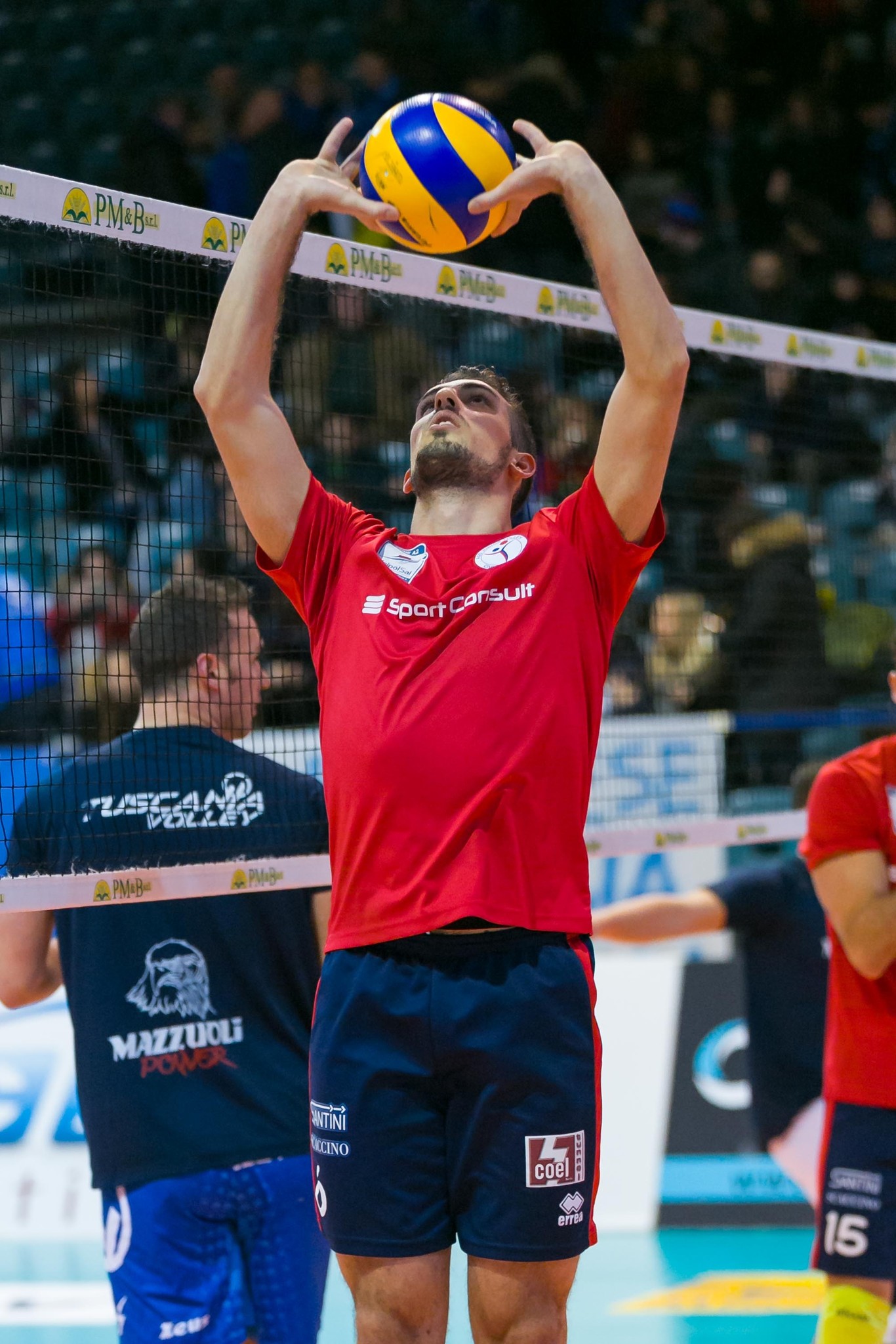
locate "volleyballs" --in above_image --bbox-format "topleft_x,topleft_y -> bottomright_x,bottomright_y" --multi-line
358,91 -> 517,256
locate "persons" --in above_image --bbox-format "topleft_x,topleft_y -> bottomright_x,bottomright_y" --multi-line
0,0 -> 896,1344
192,116 -> 688,1344
